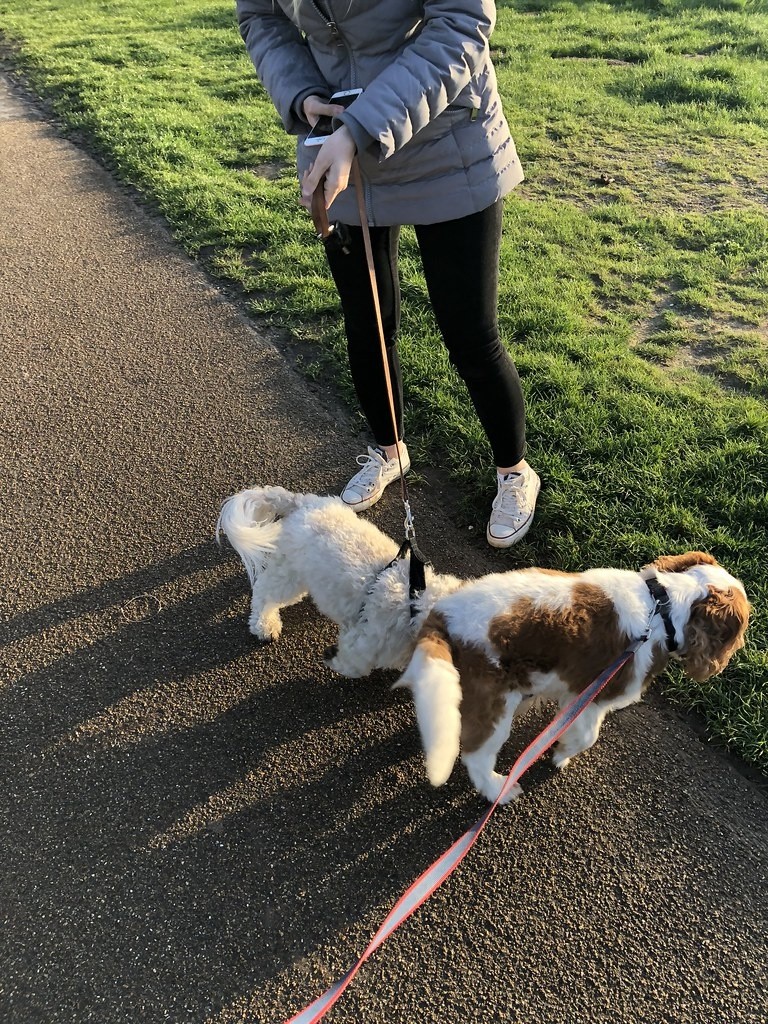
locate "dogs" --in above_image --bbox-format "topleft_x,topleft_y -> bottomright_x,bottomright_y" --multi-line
214,485 -> 464,679
388,552 -> 752,806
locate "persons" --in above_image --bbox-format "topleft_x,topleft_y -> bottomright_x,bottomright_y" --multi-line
231,0 -> 541,548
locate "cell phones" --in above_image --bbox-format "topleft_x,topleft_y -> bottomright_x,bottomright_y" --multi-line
304,88 -> 363,147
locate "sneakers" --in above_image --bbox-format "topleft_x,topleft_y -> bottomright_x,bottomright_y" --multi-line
487,464 -> 540,548
340,443 -> 410,512
